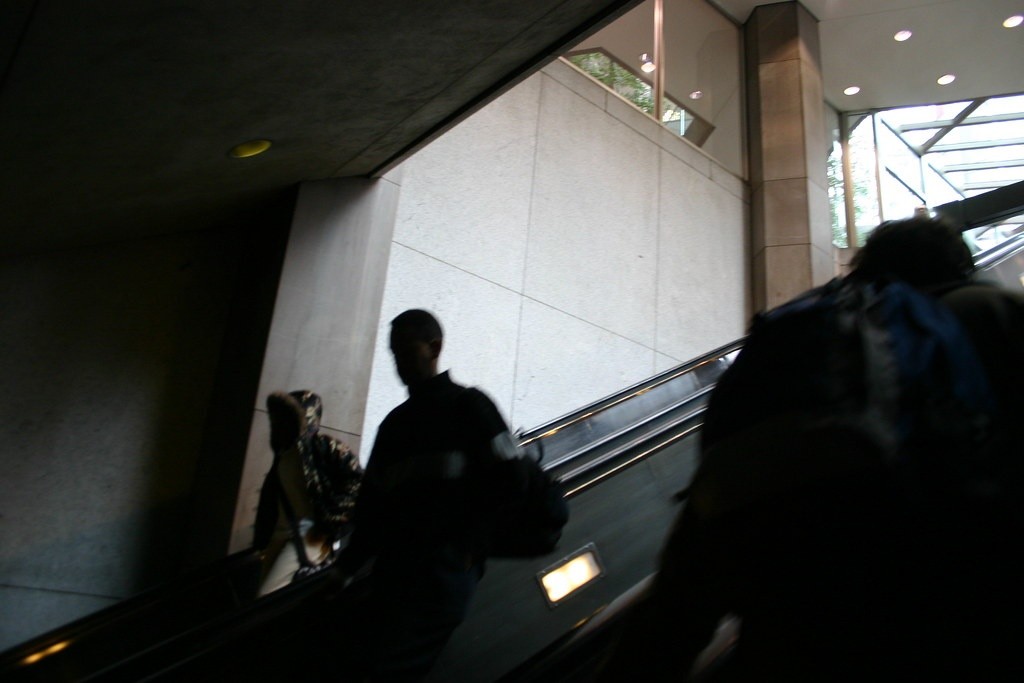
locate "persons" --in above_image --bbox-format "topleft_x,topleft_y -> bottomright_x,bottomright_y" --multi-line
327,310 -> 568,683
241,388 -> 363,605
609,220 -> 1024,683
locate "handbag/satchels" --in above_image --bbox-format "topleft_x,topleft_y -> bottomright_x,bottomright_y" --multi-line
425,452 -> 570,559
